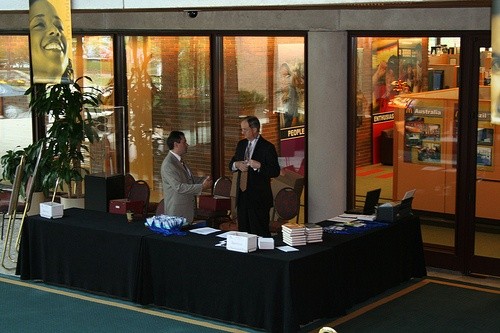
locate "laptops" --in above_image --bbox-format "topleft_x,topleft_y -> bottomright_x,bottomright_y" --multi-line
344,188 -> 381,215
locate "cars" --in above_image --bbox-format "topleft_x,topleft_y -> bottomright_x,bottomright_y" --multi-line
0,70 -> 31,119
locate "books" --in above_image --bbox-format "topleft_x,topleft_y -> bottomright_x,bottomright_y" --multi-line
227,233 -> 257,253
282,223 -> 306,245
303,223 -> 323,243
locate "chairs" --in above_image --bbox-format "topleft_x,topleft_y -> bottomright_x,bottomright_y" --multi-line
126,180 -> 150,217
270,186 -> 300,231
198,176 -> 232,228
0,174 -> 35,239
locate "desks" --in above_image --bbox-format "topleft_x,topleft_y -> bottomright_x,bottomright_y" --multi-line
15,207 -> 428,333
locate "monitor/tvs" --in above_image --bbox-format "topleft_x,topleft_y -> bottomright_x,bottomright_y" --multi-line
428,69 -> 445,90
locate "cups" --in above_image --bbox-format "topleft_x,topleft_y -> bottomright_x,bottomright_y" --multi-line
127,212 -> 134,222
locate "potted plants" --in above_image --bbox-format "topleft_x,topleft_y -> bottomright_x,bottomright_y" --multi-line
0,58 -> 103,216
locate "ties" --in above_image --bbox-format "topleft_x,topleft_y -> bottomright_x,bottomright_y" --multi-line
240,142 -> 251,192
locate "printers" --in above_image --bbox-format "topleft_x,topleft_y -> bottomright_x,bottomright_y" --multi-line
377,197 -> 414,223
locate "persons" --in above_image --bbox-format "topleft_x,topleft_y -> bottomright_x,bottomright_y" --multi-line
160,131 -> 214,225
403,58 -> 415,92
30,0 -> 68,83
372,54 -> 399,113
228,116 -> 281,238
273,63 -> 300,127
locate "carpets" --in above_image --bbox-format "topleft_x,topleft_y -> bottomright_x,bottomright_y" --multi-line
301,275 -> 500,333
0,273 -> 265,333
